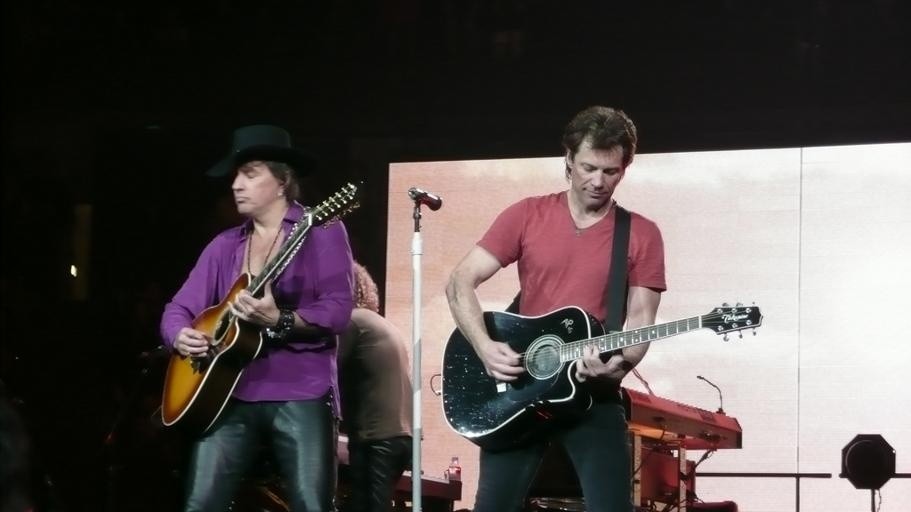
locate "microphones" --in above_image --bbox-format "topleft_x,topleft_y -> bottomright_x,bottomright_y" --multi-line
696,375 -> 726,416
408,187 -> 442,211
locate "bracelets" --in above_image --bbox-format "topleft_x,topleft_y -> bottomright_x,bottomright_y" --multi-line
272,309 -> 296,335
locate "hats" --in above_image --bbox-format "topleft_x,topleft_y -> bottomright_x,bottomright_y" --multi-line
231,124 -> 292,167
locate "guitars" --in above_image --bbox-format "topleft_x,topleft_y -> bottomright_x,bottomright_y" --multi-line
162,179 -> 365,437
442,303 -> 763,446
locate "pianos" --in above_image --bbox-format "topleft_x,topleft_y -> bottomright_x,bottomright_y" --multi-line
623,386 -> 741,438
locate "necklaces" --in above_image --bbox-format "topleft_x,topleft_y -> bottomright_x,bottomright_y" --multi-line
247,225 -> 285,278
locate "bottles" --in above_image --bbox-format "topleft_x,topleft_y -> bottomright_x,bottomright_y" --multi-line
448,455 -> 461,482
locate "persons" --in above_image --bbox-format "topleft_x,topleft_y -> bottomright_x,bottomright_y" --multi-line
335,260 -> 414,512
444,107 -> 668,512
160,123 -> 359,512
